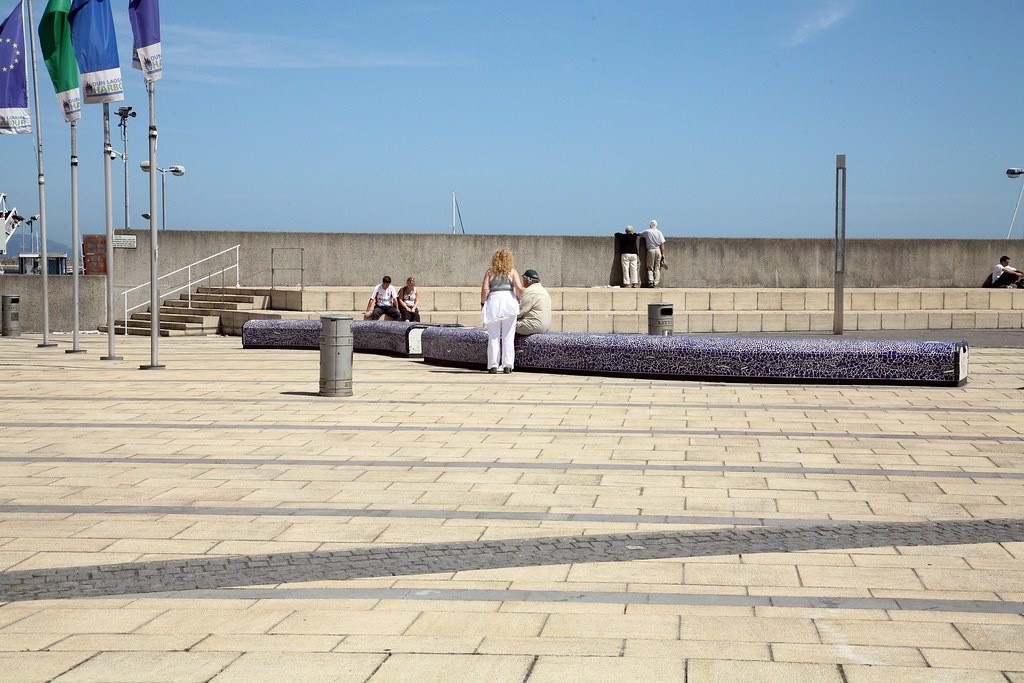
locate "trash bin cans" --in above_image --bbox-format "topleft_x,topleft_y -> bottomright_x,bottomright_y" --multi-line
2,295 -> 21,337
319,314 -> 354,397
648,302 -> 674,336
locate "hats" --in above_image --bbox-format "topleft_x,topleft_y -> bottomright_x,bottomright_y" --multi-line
661,259 -> 668,270
521,269 -> 538,279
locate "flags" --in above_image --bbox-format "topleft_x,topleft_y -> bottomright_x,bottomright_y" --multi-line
67,0 -> 124,104
127,0 -> 163,95
38,0 -> 82,123
0,0 -> 32,135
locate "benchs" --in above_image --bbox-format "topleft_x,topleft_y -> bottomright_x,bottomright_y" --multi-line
419,326 -> 971,389
240,319 -> 465,359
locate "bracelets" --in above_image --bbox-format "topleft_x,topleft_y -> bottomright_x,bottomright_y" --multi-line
481,302 -> 484,306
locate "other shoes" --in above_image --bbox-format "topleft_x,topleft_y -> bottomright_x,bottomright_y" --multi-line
488,367 -> 512,373
649,283 -> 659,288
623,283 -> 640,288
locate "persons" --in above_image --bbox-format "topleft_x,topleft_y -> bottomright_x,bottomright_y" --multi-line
397,277 -> 420,323
365,275 -> 400,321
480,248 -> 522,374
991,255 -> 1024,289
30,261 -> 39,274
638,220 -> 666,288
615,226 -> 641,288
498,269 -> 552,370
